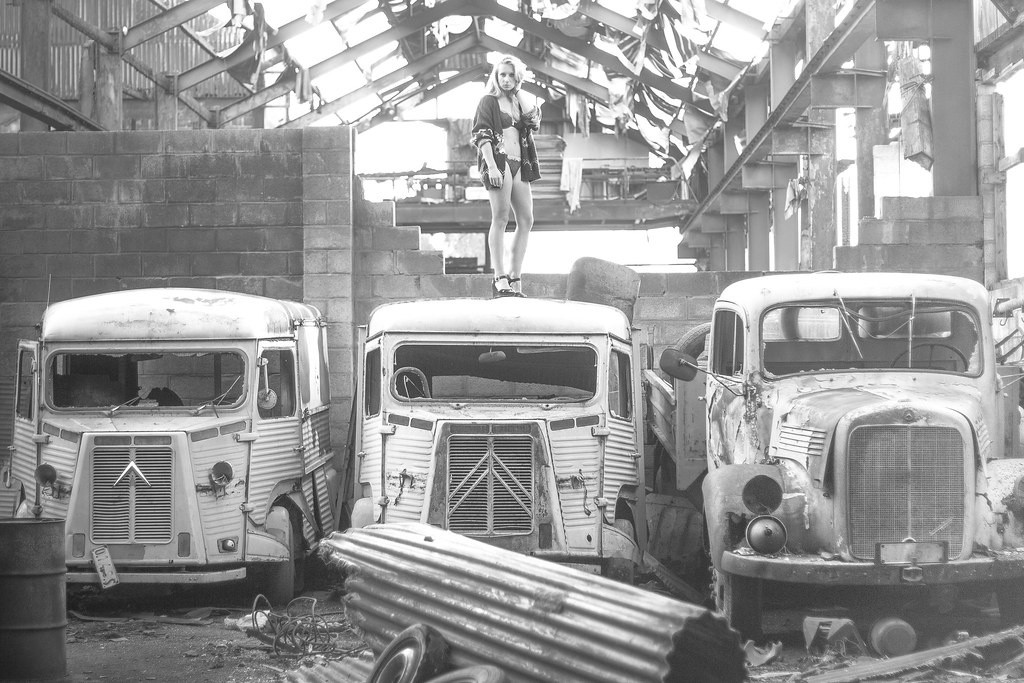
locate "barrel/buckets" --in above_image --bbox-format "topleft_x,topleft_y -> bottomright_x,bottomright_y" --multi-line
0,518 -> 67,683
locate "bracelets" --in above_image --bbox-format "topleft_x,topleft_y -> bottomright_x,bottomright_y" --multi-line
514,89 -> 519,96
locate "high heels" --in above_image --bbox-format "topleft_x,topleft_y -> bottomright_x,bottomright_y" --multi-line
491,274 -> 528,299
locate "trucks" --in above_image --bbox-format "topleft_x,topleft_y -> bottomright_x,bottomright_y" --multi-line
4,287 -> 337,617
352,296 -> 646,584
642,272 -> 1024,640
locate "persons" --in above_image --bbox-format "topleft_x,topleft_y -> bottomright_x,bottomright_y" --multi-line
471,52 -> 541,298
